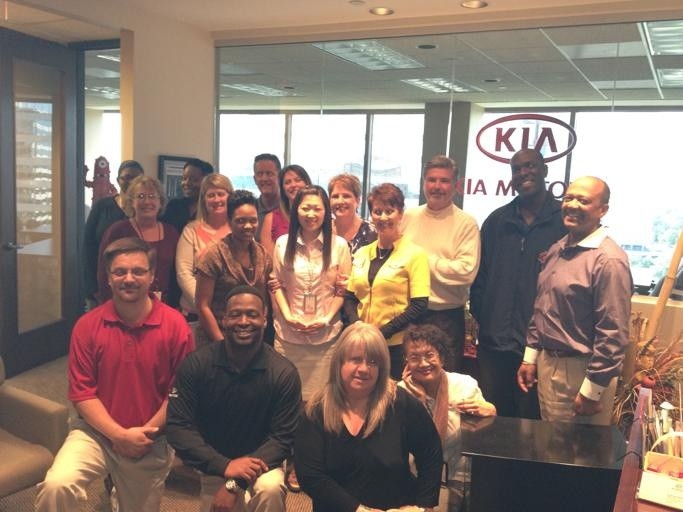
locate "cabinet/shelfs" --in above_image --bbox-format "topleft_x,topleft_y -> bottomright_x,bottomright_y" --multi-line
460,417 -> 627,512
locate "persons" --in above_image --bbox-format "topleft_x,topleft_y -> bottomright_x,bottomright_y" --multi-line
81,154 -> 378,347
469,147 -> 568,419
396,324 -> 497,512
340,183 -> 431,383
267,186 -> 352,494
34,237 -> 197,512
165,283 -> 304,512
515,174 -> 635,425
295,320 -> 444,512
400,156 -> 481,373
652,265 -> 683,300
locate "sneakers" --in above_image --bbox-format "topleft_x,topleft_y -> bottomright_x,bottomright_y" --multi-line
287,468 -> 302,490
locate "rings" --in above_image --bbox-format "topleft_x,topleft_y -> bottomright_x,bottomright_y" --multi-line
471,412 -> 474,415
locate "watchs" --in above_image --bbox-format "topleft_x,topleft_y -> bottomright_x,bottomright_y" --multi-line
225,481 -> 241,496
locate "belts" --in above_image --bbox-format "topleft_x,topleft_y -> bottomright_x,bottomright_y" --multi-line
542,347 -> 581,357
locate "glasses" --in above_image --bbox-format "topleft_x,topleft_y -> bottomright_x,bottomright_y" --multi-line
133,193 -> 160,200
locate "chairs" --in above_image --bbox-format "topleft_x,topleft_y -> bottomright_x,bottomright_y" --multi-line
0,355 -> 71,512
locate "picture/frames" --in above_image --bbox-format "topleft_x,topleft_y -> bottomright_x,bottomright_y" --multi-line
159,154 -> 197,199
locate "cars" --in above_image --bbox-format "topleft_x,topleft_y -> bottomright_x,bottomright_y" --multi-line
619,243 -> 660,258
